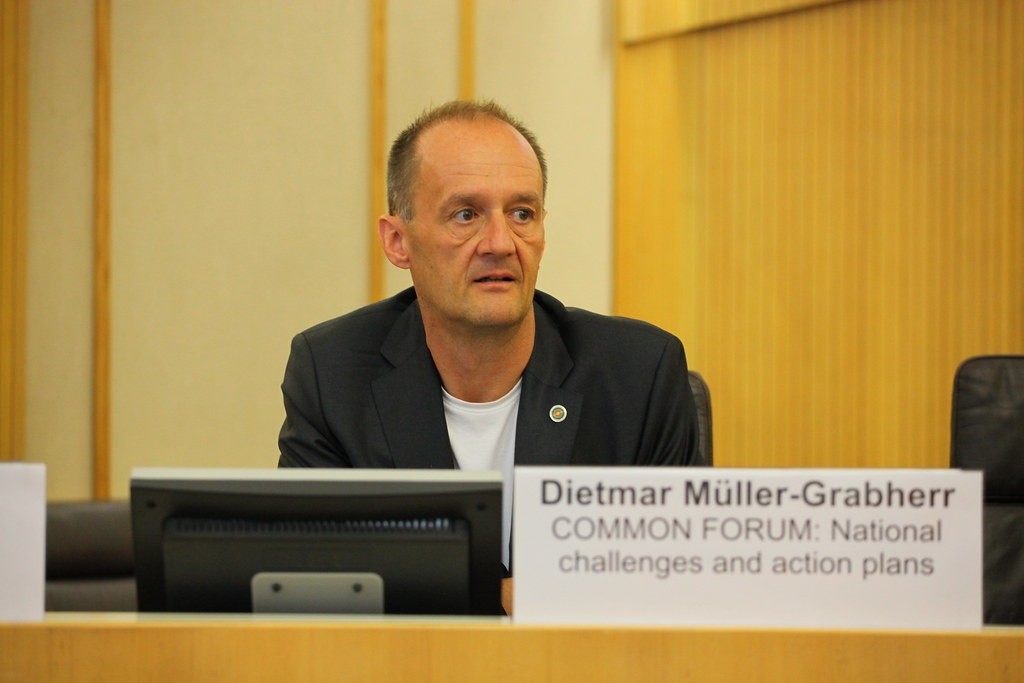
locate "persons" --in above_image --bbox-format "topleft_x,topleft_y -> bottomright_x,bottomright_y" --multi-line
274,100 -> 703,620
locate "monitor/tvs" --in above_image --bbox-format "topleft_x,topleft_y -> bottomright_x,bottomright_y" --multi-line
130,468 -> 505,617
